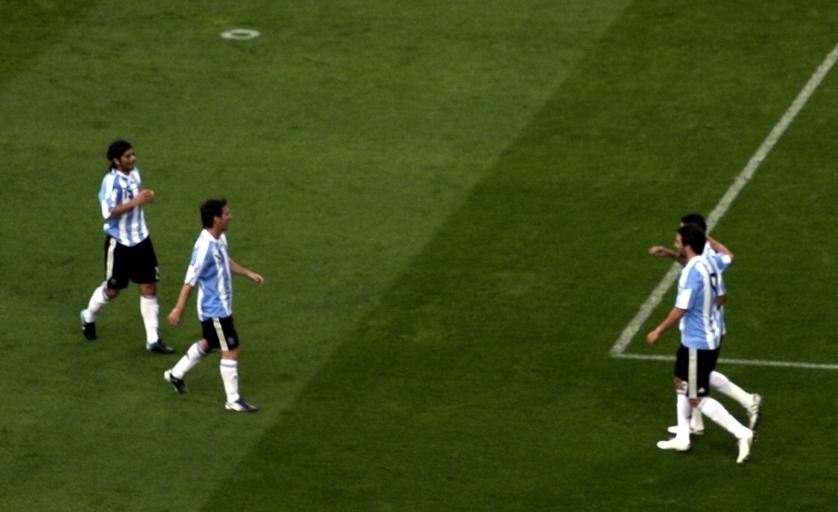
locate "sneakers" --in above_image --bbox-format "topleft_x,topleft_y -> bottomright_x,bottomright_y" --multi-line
81,309 -> 97,339
668,424 -> 704,437
746,391 -> 761,430
225,398 -> 258,412
146,339 -> 174,352
163,369 -> 188,393
737,430 -> 757,465
657,440 -> 691,452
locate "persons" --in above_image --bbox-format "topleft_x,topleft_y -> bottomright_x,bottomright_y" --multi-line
80,140 -> 175,354
648,213 -> 762,436
647,225 -> 757,464
162,199 -> 264,413
